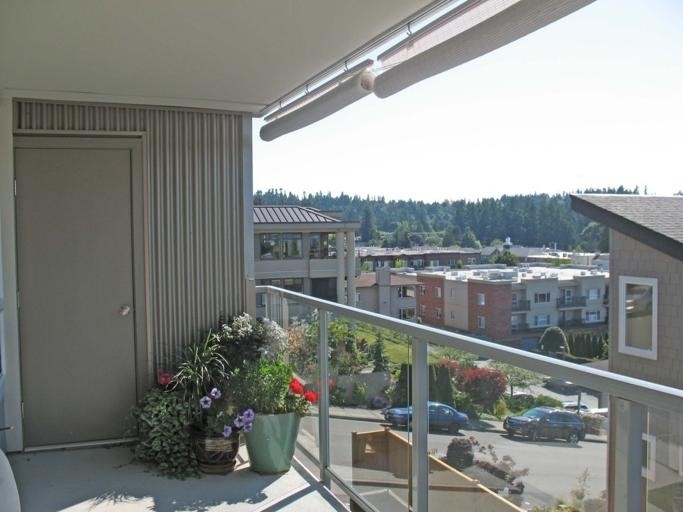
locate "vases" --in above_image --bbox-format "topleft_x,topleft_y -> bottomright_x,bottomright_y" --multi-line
189,412 -> 301,474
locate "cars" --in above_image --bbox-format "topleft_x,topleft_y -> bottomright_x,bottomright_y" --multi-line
503,406 -> 586,444
384,400 -> 470,434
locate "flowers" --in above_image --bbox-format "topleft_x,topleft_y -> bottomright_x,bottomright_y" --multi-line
157,311 -> 319,436
